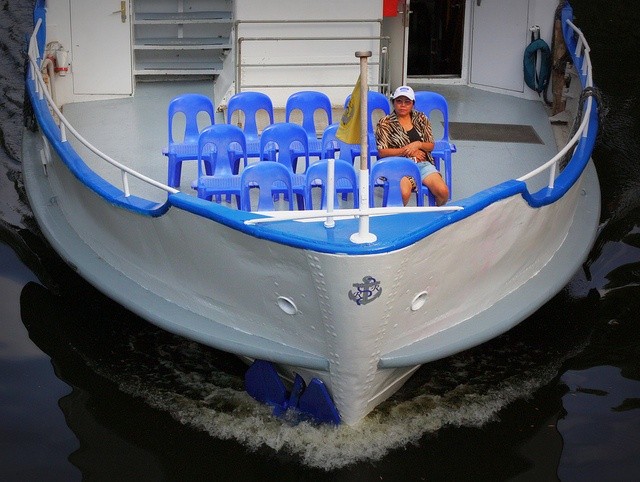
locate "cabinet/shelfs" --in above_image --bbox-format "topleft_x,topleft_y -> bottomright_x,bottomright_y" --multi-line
131,0 -> 235,97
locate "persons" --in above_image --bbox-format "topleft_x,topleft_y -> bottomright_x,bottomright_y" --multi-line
374,86 -> 449,206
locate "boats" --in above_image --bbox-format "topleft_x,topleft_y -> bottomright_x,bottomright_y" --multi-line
21,0 -> 603,427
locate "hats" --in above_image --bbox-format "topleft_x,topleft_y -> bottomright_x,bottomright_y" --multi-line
393,86 -> 415,101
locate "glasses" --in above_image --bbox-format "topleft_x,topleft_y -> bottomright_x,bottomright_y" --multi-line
396,99 -> 411,105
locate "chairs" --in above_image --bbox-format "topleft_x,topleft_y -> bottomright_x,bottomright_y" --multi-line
369,156 -> 424,207
413,91 -> 457,201
304,158 -> 359,210
252,122 -> 315,210
315,123 -> 378,209
161,93 -> 222,204
240,160 -> 293,211
227,91 -> 281,203
340,90 -> 391,202
190,123 -> 254,211
282,90 -> 333,201
422,184 -> 436,206
